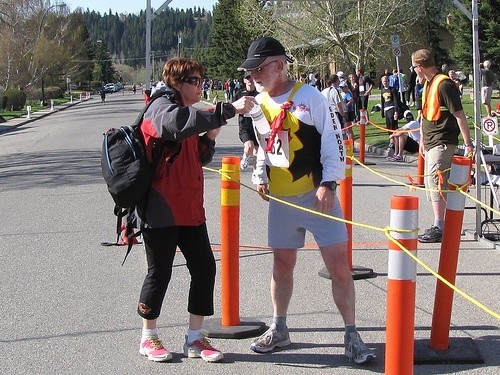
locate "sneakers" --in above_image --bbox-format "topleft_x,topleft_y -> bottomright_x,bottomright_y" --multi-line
344,330 -> 377,364
417,224 -> 442,244
139,336 -> 173,362
249,322 -> 292,354
182,334 -> 224,362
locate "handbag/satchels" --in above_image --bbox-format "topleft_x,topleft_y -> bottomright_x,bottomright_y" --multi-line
396,104 -> 410,121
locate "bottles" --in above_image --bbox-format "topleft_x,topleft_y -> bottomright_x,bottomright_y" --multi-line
248,103 -> 271,134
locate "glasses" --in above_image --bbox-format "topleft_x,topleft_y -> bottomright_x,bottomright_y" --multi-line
409,65 -> 417,73
245,60 -> 278,75
178,76 -> 203,86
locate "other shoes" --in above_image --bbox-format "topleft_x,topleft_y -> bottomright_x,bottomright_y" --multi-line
387,154 -> 403,162
240,153 -> 250,171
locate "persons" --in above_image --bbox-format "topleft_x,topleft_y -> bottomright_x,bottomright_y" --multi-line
143,79 -> 167,107
299,64 -> 464,148
231,70 -> 261,184
389,110 -> 421,163
411,48 -> 474,244
479,60 -> 495,116
100,89 -> 105,104
201,73 -> 245,107
135,56 -> 259,362
132,83 -> 136,95
238,37 -> 376,365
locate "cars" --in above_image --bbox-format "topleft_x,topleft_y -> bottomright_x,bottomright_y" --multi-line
103,82 -> 124,93
212,81 -> 222,90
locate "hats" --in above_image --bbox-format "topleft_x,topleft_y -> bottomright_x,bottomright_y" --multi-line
244,72 -> 250,77
237,37 -> 294,72
403,110 -> 413,119
484,61 -> 491,67
335,71 -> 344,77
338,82 -> 348,88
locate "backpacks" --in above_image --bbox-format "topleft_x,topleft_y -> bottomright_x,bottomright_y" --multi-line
101,90 -> 186,208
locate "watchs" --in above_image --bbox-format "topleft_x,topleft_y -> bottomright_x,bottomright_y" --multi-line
320,180 -> 338,191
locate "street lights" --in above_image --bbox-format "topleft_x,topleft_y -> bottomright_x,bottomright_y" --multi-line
40,3 -> 66,107
